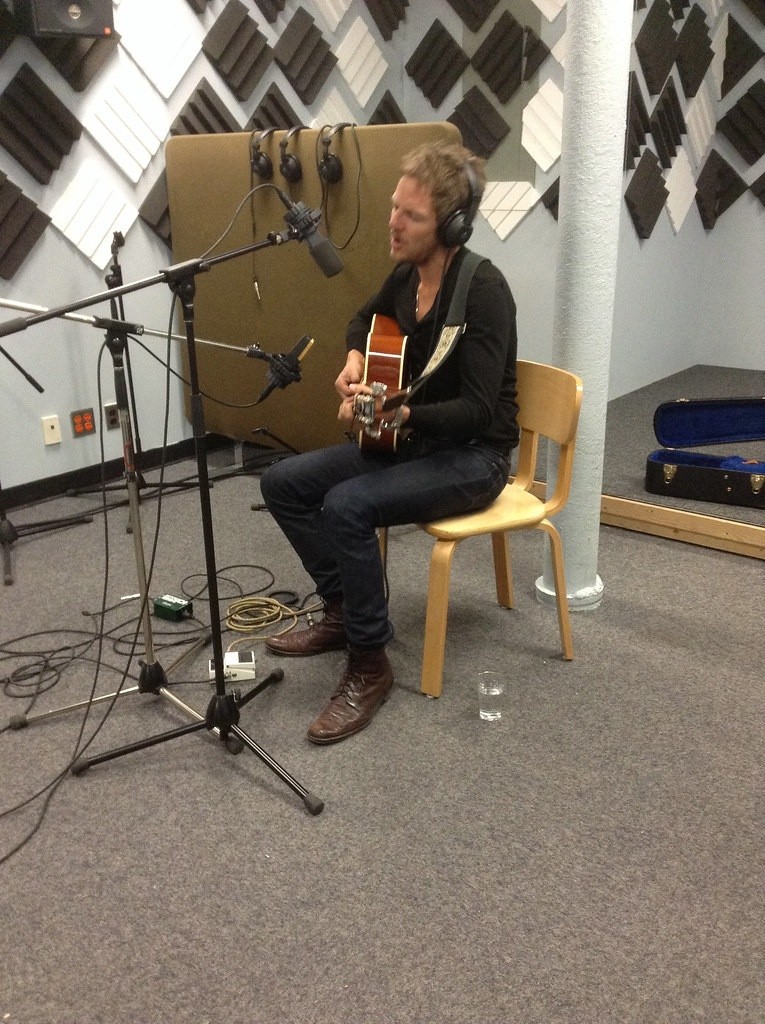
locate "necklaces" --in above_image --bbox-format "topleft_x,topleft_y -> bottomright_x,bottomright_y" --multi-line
415,282 -> 422,320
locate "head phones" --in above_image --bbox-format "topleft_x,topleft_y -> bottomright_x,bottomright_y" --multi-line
247,122 -> 351,185
439,160 -> 483,249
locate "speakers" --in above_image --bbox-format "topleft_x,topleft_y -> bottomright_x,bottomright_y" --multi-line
13,1 -> 113,36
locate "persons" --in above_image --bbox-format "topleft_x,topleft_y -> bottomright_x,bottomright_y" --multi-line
262,140 -> 519,744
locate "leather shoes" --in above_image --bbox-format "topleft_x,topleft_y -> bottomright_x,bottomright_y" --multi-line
306,647 -> 395,746
265,597 -> 344,655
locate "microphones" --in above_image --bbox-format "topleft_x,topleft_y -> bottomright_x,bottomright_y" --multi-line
275,189 -> 345,279
255,334 -> 317,405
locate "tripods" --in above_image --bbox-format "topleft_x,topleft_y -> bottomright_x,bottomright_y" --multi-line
0,227 -> 326,818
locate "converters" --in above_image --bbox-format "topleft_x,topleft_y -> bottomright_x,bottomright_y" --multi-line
153,594 -> 194,624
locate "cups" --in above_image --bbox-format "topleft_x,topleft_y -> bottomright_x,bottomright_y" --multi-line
477,670 -> 508,721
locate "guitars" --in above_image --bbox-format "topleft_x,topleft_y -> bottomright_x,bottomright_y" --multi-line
348,314 -> 410,453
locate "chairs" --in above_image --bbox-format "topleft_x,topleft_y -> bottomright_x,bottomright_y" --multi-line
379,360 -> 582,699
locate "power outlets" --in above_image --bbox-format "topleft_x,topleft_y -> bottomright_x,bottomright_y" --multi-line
42,415 -> 61,446
70,408 -> 96,438
104,403 -> 121,432
